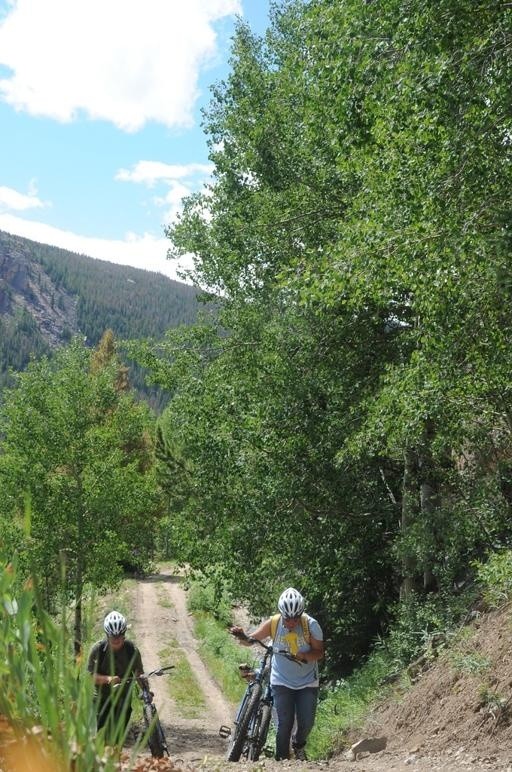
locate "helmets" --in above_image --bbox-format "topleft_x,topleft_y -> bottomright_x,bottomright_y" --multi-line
103,611 -> 127,638
277,587 -> 305,619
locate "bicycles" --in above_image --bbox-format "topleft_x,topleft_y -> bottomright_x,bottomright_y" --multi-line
220,629 -> 309,762
110,663 -> 177,761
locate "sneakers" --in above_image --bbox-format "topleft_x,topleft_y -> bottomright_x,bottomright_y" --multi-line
294,745 -> 307,761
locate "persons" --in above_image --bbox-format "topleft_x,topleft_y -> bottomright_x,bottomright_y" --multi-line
89,611 -> 148,746
241,640 -> 297,760
228,588 -> 324,762
